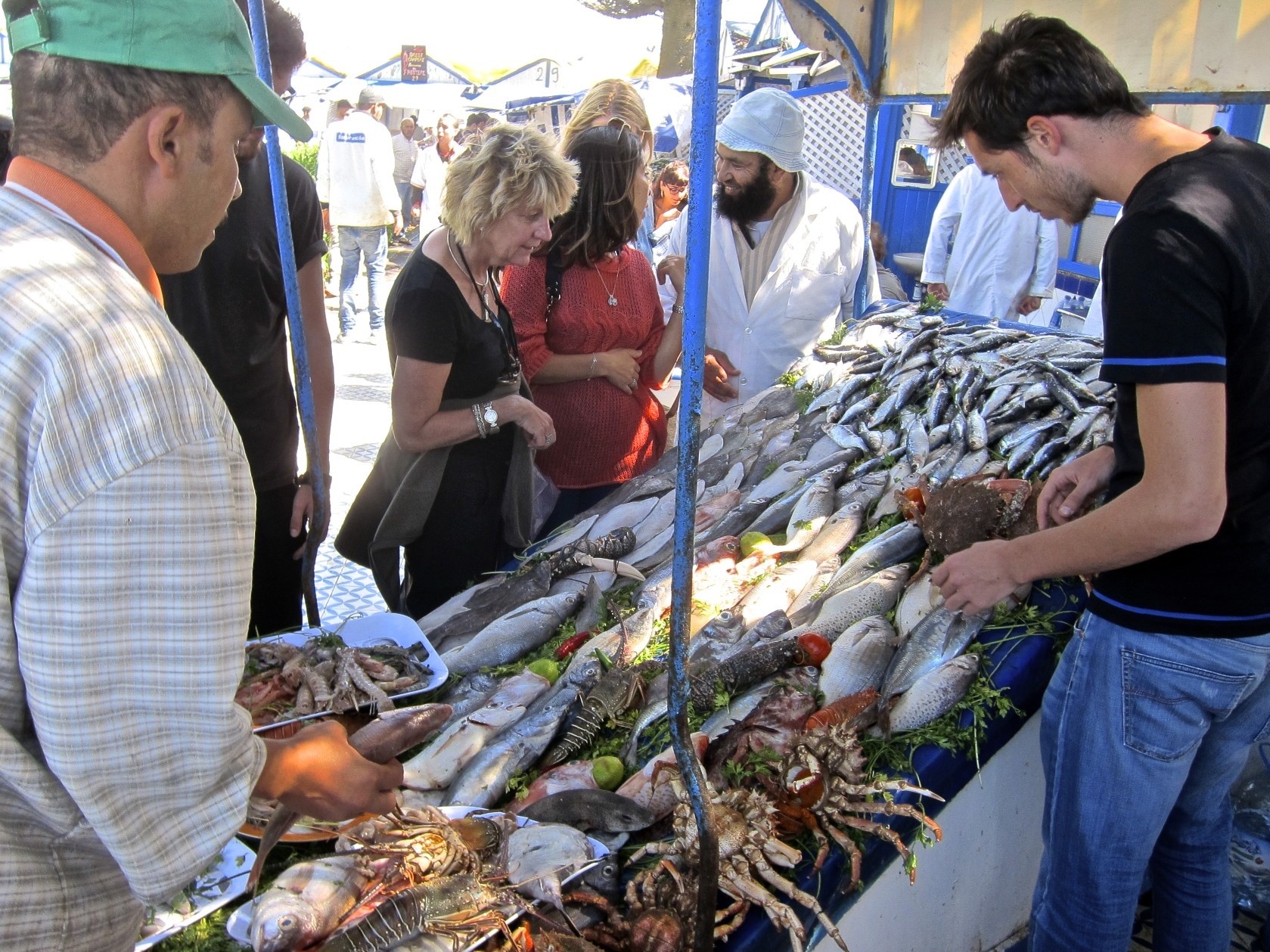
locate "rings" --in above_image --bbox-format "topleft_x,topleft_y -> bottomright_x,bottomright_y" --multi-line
630,379 -> 637,388
546,434 -> 552,442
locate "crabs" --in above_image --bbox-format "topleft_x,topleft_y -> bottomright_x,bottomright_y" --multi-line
892,473 -> 1032,587
554,856 -> 750,952
622,749 -> 852,952
749,682 -> 945,896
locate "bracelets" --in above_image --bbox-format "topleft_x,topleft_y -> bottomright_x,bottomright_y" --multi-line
588,353 -> 597,382
297,469 -> 333,488
673,304 -> 683,314
472,404 -> 486,439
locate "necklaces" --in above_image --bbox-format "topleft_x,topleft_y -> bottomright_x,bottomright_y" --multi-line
595,251 -> 619,306
447,227 -> 489,320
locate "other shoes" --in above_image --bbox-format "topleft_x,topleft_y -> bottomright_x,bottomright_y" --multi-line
399,236 -> 413,245
325,285 -> 340,297
369,329 -> 387,345
336,332 -> 357,344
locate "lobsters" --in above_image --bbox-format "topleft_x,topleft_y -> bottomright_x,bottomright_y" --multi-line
311,804 -> 575,952
540,595 -> 667,767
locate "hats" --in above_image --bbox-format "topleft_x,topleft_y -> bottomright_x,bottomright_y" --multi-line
2,0 -> 313,142
716,87 -> 810,172
359,89 -> 393,111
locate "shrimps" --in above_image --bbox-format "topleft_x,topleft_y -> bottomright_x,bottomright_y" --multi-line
233,634 -> 430,714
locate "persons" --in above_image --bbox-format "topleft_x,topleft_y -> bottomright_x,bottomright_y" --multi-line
316,86 -> 499,343
301,106 -> 318,138
870,222 -> 910,302
651,161 -> 689,230
155,0 -> 335,640
920,163 -> 1058,322
381,122 -> 579,622
900,147 -> 932,175
563,78 -> 658,265
929,13 -> 1270,952
498,127 -> 685,542
0,0 -> 404,952
656,87 -> 882,449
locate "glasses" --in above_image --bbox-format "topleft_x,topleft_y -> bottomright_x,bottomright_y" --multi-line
281,86 -> 297,107
665,182 -> 686,194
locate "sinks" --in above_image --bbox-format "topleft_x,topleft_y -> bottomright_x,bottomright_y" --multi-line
892,252 -> 950,276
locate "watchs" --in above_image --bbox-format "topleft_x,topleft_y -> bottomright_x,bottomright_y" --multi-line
483,401 -> 500,435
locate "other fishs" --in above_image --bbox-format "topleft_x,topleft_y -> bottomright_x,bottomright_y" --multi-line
423,560 -> 552,651
392,697 -> 525,790
498,820 -> 596,936
247,851 -> 372,952
530,857 -> 620,936
436,702 -> 570,808
513,787 -> 655,833
505,756 -> 597,816
613,731 -> 712,819
244,700 -> 453,896
443,590 -> 582,676
531,297 -> 1118,744
